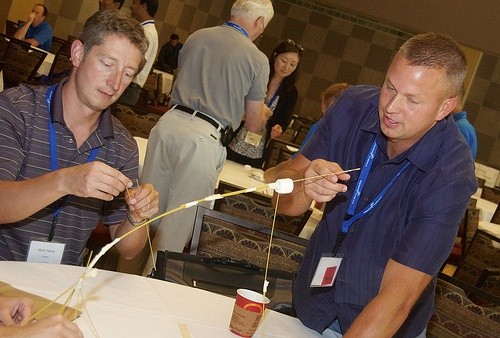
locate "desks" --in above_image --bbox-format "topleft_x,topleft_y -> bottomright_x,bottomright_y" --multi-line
131,137 -> 275,200
1,259 -> 318,338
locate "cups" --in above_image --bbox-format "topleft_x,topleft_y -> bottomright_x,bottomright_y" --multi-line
228,289 -> 270,338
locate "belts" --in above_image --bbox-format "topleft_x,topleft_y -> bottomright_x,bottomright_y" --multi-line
170,104 -> 224,134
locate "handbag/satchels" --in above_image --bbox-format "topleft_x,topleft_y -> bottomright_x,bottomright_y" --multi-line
147,250 -> 298,318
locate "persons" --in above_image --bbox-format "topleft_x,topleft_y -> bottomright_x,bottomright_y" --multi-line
158,33 -> 183,73
99,0 -> 183,108
99,0 -> 125,10
0,10 -> 158,260
289,83 -> 352,162
448,82 -> 477,162
116,0 -> 273,277
13,3 -> 53,52
226,38 -> 303,170
271,33 -> 477,338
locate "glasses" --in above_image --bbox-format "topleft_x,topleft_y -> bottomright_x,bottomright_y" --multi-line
286,38 -> 305,51
260,21 -> 266,37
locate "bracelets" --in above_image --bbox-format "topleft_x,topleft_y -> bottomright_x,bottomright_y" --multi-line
127,208 -> 149,227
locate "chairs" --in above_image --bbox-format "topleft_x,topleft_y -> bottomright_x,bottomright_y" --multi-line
0,20 -> 171,110
426,198 -> 500,338
151,181 -> 313,300
